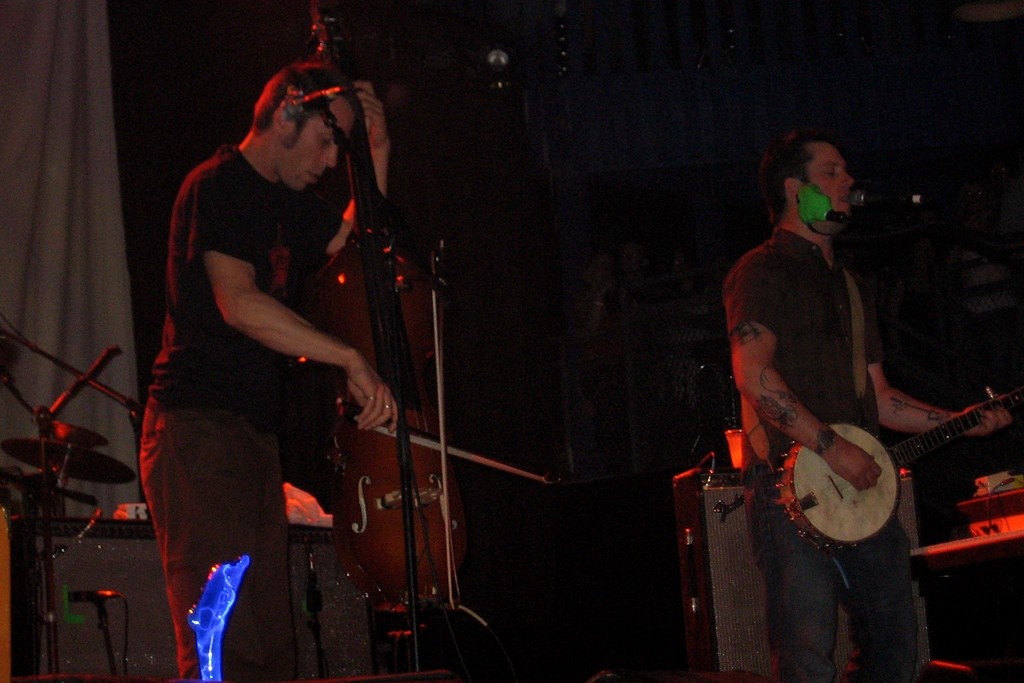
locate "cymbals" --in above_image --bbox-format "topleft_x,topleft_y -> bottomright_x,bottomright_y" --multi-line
0,436 -> 136,484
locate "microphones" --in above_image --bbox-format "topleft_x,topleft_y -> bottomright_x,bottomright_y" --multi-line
381,486 -> 444,510
284,85 -> 341,119
68,590 -> 123,604
848,188 -> 930,205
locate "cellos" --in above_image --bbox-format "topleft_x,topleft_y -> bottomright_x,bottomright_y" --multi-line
296,0 -> 474,675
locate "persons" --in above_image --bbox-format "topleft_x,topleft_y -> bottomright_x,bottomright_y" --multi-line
718,122 -> 1013,682
134,60 -> 402,683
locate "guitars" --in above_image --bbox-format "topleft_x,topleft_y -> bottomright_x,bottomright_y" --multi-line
771,384 -> 1024,554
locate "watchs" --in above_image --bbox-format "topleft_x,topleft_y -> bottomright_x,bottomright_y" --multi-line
813,428 -> 836,456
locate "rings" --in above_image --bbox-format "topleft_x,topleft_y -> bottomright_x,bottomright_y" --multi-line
367,395 -> 376,400
384,403 -> 393,410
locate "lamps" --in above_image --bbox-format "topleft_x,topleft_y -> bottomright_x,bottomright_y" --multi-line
464,48 -> 511,99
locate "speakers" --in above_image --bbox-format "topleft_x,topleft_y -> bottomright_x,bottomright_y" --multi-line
672,465 -> 929,683
8,514 -> 180,680
288,523 -> 377,678
